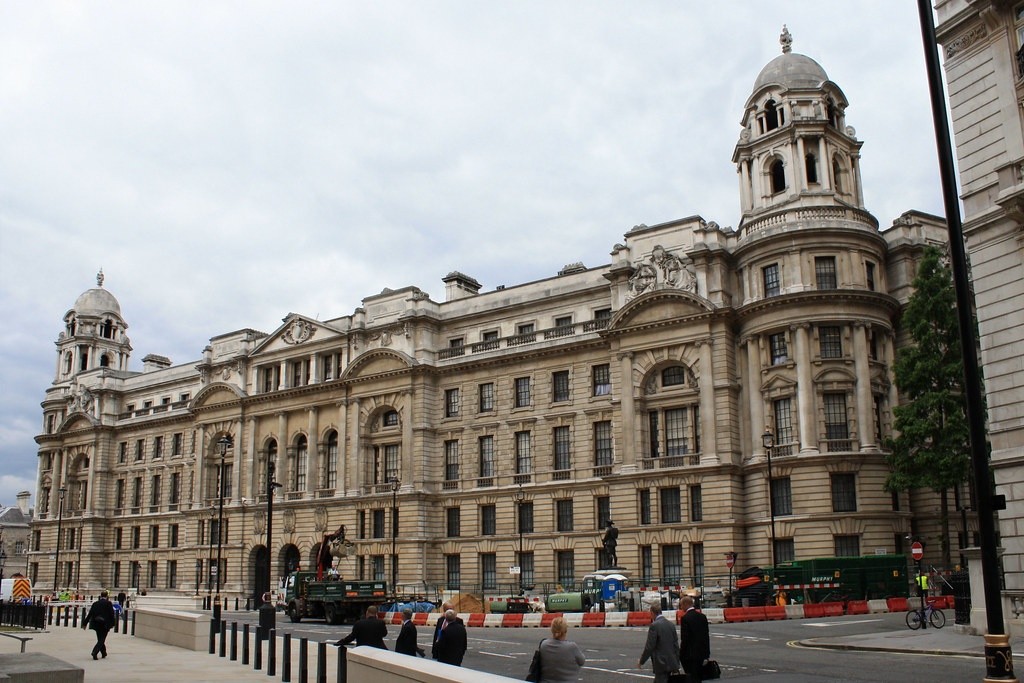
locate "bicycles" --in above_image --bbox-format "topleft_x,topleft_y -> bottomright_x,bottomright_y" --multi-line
905,599 -> 946,630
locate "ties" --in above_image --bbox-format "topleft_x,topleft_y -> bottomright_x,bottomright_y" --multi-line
443,621 -> 446,630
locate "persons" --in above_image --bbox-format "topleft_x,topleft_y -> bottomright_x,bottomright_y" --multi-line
394,608 -> 426,658
916,572 -> 929,611
333,605 -> 388,650
776,590 -> 786,607
637,603 -> 681,683
537,618 -> 585,683
602,519 -> 618,567
45,589 -> 71,603
261,590 -> 271,605
82,590 -> 115,660
432,602 -> 464,659
439,609 -> 467,667
113,589 -> 146,616
680,596 -> 710,683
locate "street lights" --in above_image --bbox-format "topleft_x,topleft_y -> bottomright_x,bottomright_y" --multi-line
516,486 -> 526,596
390,477 -> 400,599
212,435 -> 232,635
760,428 -> 777,573
50,486 -> 68,602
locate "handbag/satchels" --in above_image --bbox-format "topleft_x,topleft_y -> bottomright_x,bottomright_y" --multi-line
95,616 -> 104,625
525,638 -> 547,683
668,669 -> 689,683
701,658 -> 721,680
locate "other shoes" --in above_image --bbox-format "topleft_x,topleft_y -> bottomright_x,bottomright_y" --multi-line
102,653 -> 107,658
92,653 -> 98,660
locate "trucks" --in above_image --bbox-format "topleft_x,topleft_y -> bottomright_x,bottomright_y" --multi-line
760,553 -> 910,604
283,569 -> 387,625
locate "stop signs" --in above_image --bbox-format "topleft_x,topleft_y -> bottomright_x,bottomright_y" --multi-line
726,556 -> 735,568
911,541 -> 923,560
